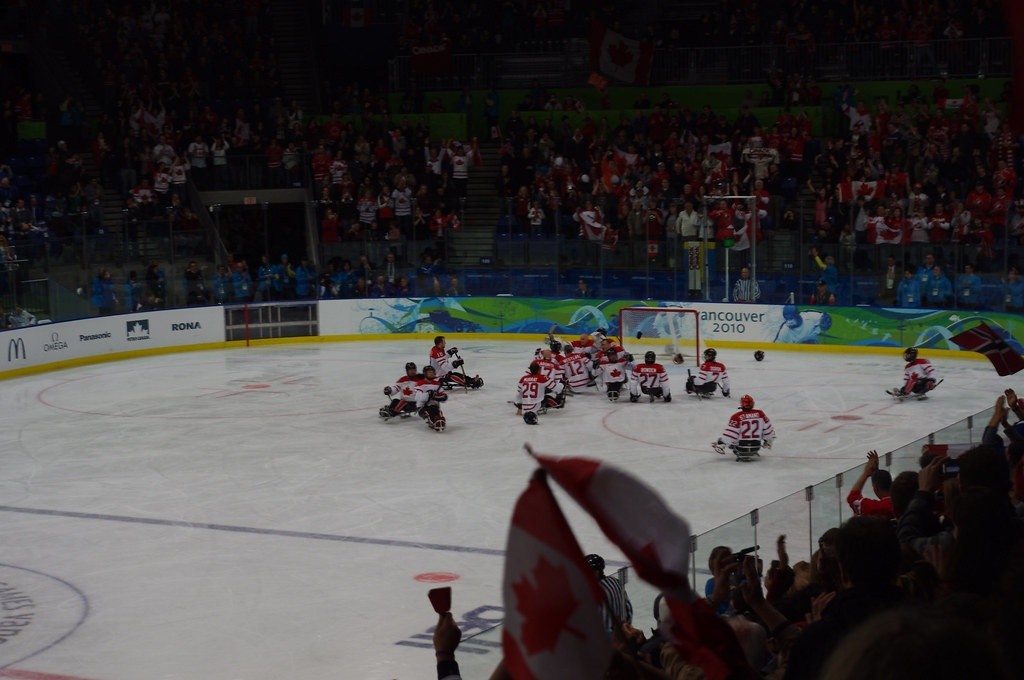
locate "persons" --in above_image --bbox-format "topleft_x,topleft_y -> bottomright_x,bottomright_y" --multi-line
432,389 -> 1024,680
0,0 -> 1024,327
712,395 -> 778,463
511,328 -> 672,414
377,361 -> 448,430
687,349 -> 731,398
430,336 -> 482,389
886,347 -> 942,400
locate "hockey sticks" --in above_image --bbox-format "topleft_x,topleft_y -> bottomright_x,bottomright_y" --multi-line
548,324 -> 558,341
386,394 -> 393,401
454,353 -> 467,394
419,382 -> 444,415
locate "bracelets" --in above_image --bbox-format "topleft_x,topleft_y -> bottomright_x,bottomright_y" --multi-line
435,650 -> 456,659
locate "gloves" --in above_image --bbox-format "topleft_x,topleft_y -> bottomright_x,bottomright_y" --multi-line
629,392 -> 641,402
452,357 -> 464,368
447,347 -> 458,357
664,393 -> 671,402
384,386 -> 392,396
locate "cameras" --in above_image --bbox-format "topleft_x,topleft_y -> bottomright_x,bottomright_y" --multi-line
942,459 -> 960,476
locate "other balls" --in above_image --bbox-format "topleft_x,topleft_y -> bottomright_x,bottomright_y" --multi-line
637,332 -> 642,339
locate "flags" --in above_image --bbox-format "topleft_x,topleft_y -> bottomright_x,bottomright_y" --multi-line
841,180 -> 886,202
704,143 -> 732,164
614,148 -> 642,170
592,25 -> 650,83
867,222 -> 914,248
716,222 -> 757,252
580,210 -> 608,244
502,469 -> 615,680
341,7 -> 373,28
524,444 -> 757,680
936,98 -> 970,111
949,320 -> 1024,377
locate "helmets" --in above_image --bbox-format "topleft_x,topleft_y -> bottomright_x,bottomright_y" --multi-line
585,554 -> 606,572
607,348 -> 617,358
523,411 -> 538,425
703,348 -> 717,361
740,394 -> 754,408
644,351 -> 656,364
423,365 -> 436,372
903,347 -> 918,362
597,327 -> 608,337
404,362 -> 417,370
550,341 -> 562,351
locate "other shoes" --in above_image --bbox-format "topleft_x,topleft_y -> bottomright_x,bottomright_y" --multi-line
379,405 -> 396,417
432,415 -> 446,432
917,394 -> 929,401
606,388 -> 620,401
